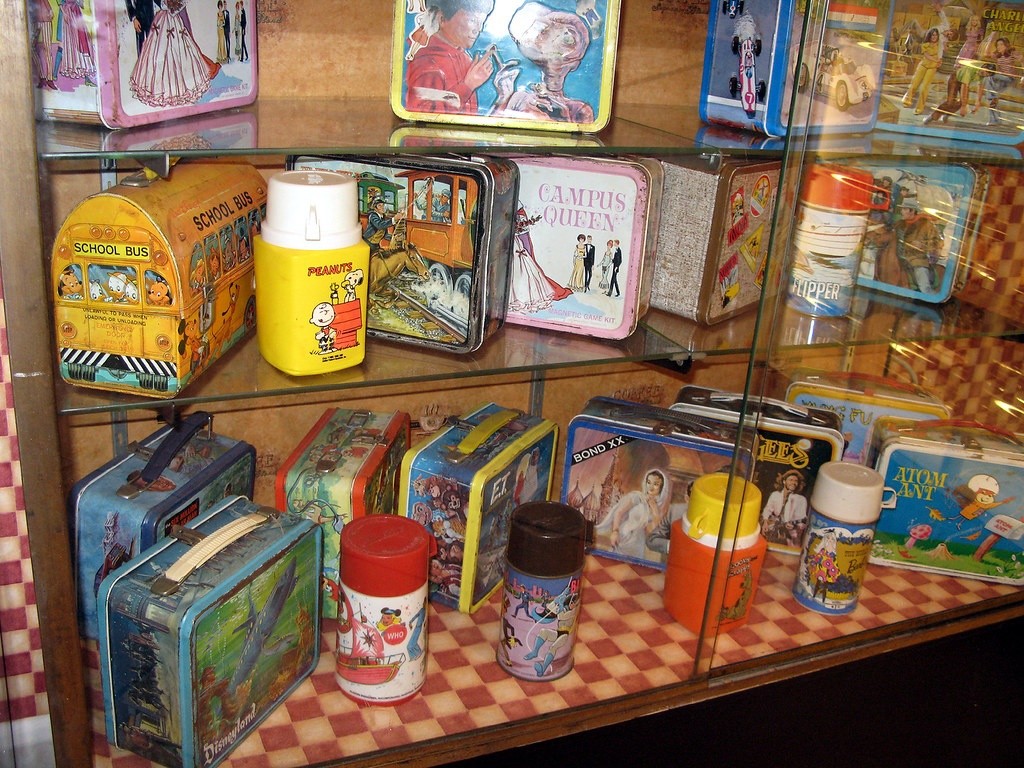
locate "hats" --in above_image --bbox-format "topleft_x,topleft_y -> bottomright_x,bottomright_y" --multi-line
779,471 -> 804,492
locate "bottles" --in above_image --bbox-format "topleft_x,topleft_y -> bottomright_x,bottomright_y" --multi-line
666,476 -> 766,635
337,513 -> 438,707
794,462 -> 896,613
787,161 -> 889,319
254,168 -> 371,378
498,499 -> 588,682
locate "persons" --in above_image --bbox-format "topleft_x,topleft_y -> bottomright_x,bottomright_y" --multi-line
761,471 -> 810,547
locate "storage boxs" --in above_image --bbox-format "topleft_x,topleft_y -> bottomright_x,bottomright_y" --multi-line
26,0 -> 1024,768
